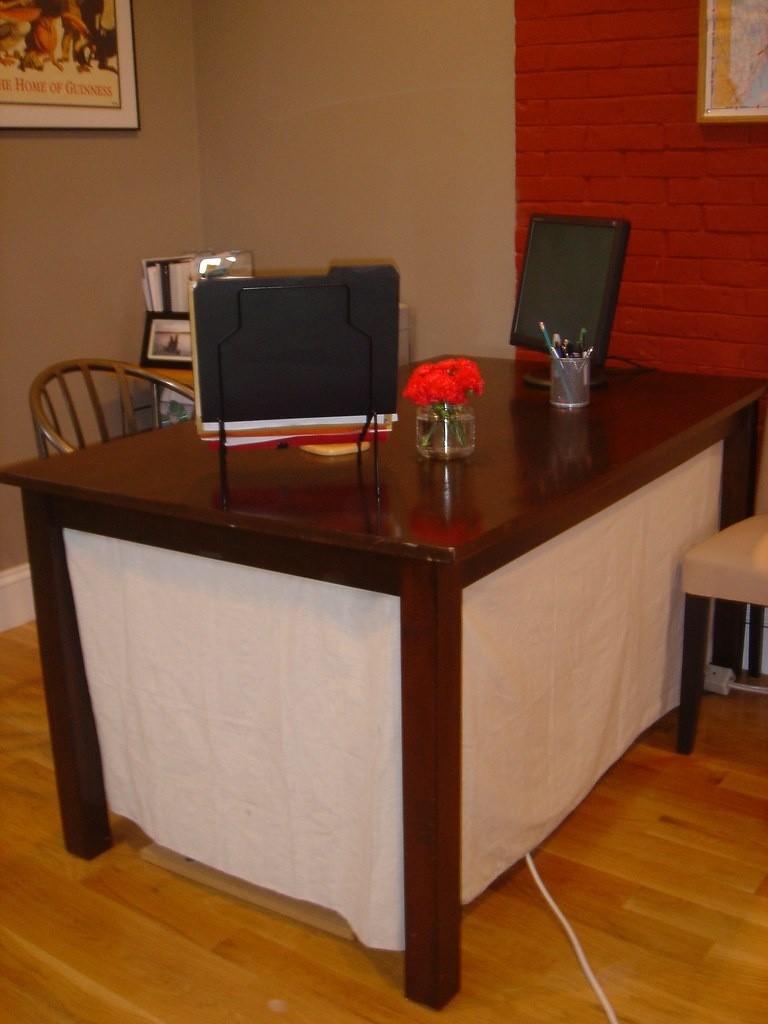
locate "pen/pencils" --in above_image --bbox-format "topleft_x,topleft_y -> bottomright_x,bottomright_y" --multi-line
537,320 -> 596,403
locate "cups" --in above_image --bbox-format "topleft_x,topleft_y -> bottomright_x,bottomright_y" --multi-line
549,356 -> 590,408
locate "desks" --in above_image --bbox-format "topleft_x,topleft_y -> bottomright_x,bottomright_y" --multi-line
0,355 -> 768,1013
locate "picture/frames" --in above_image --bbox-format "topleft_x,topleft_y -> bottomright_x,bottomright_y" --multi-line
696,0 -> 768,123
139,311 -> 193,371
0,0 -> 141,131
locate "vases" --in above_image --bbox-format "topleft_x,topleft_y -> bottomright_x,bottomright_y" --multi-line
416,405 -> 476,460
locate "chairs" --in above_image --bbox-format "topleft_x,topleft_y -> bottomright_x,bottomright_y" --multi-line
676,407 -> 768,754
29,358 -> 195,459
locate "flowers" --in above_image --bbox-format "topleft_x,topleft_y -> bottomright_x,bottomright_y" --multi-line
402,357 -> 482,447
